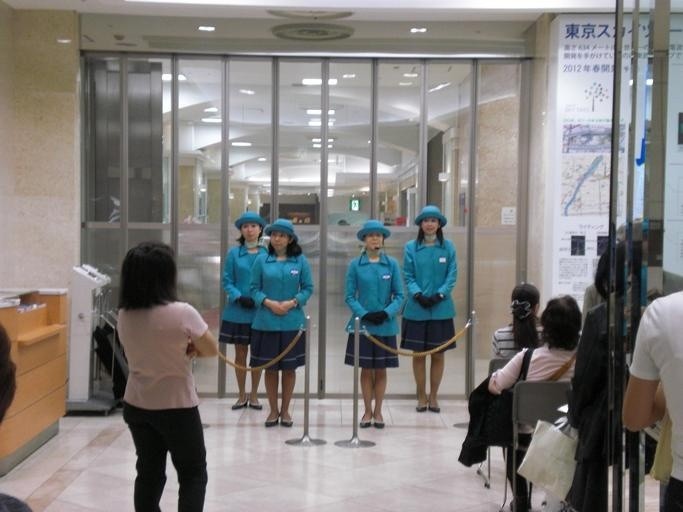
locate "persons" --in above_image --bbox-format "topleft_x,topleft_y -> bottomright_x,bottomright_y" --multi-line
0,322 -> 34,512
489,241 -> 683,512
343,220 -> 404,429
116,240 -> 218,512
218,211 -> 269,409
248,218 -> 314,427
399,204 -> 458,413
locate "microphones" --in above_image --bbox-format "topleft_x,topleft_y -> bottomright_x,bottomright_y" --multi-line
374,238 -> 384,249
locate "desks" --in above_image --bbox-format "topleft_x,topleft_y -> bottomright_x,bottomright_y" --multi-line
0,288 -> 71,478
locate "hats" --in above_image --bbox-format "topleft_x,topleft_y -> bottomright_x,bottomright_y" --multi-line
414,205 -> 447,228
265,218 -> 299,241
356,220 -> 391,241
235,211 -> 267,229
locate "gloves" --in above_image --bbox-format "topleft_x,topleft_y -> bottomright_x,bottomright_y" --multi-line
238,296 -> 255,309
364,311 -> 388,325
416,293 -> 442,308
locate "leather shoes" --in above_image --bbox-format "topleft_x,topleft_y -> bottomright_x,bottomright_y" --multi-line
373,413 -> 384,428
360,410 -> 372,428
232,397 -> 248,409
416,395 -> 427,412
429,396 -> 440,412
265,411 -> 279,426
280,412 -> 293,427
249,398 -> 262,410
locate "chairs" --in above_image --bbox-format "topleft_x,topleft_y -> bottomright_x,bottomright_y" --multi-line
458,359 -> 511,489
499,381 -> 573,512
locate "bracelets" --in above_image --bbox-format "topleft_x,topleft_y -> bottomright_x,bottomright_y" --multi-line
293,299 -> 298,308
438,292 -> 445,300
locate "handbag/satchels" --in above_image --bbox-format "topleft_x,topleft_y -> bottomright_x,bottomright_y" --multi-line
487,390 -> 514,447
517,421 -> 579,511
